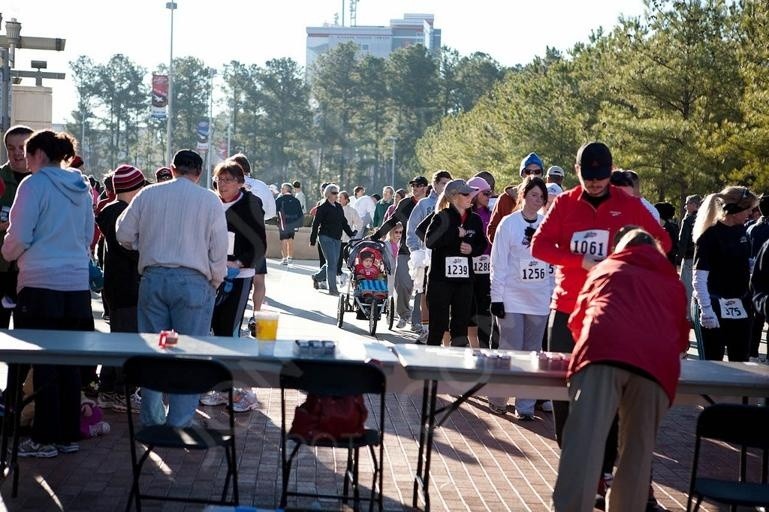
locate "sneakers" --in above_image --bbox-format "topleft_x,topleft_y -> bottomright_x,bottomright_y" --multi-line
517,414 -> 534,421
281,256 -> 429,343
536,401 -> 554,411
7,385 -> 258,458
489,401 -> 507,416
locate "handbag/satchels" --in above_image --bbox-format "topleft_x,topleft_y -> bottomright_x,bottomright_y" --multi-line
287,394 -> 369,442
89,256 -> 104,293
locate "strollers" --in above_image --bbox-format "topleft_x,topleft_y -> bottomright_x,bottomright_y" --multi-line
336,238 -> 396,334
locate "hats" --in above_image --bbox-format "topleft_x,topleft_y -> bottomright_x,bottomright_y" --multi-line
156,167 -> 173,178
361,251 -> 375,261
112,165 -> 146,194
397,152 -> 564,200
656,202 -> 675,219
575,143 -> 612,181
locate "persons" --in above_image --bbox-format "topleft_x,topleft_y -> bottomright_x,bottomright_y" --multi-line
658,185 -> 769,360
313,153 -> 563,347
275,181 -> 306,264
97,149 -> 277,426
1,125 -> 102,456
489,176 -> 553,419
532,142 -> 689,512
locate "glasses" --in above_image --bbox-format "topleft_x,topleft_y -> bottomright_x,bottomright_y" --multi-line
738,188 -> 749,204
395,231 -> 403,234
159,176 -> 171,180
331,192 -> 339,196
212,176 -> 238,184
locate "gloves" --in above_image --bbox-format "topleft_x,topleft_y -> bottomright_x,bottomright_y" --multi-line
216,267 -> 240,307
700,305 -> 721,330
490,302 -> 505,319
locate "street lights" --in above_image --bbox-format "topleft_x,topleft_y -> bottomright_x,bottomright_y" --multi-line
163,0 -> 179,168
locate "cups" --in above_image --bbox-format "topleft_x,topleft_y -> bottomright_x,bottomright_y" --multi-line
254,311 -> 278,359
